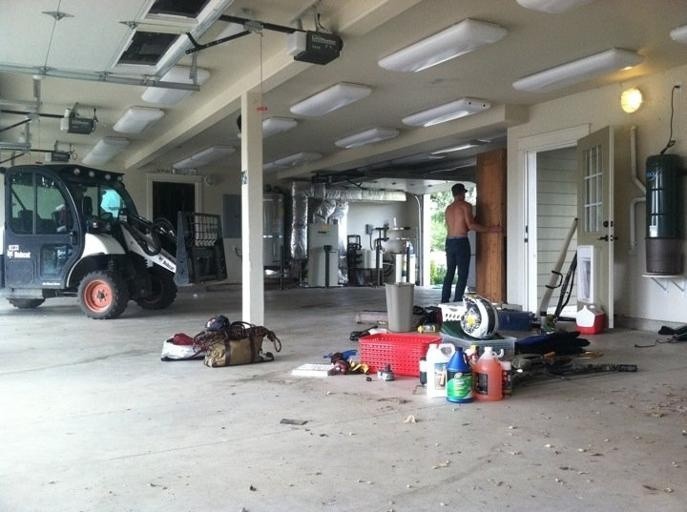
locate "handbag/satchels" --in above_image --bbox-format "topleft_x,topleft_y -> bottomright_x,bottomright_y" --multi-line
204,326 -> 274,368
161,336 -> 206,360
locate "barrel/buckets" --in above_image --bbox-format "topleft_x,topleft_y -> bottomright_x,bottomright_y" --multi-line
419,343 -> 512,404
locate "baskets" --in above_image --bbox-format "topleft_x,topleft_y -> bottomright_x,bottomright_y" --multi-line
439,331 -> 518,361
437,300 -> 502,322
358,333 -> 443,377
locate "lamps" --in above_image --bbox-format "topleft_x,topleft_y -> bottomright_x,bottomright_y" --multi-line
81,136 -> 130,169
377,17 -> 509,75
235,115 -> 299,143
287,80 -> 374,118
333,126 -> 399,151
140,65 -> 211,110
512,47 -> 646,96
401,98 -> 493,129
112,105 -> 164,137
170,144 -> 238,171
264,149 -> 323,174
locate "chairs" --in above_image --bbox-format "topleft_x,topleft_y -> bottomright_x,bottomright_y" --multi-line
18,210 -> 47,234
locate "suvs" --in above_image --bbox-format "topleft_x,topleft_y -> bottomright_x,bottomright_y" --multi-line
0,164 -> 179,319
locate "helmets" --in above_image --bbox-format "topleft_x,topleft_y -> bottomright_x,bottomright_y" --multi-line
460,293 -> 500,339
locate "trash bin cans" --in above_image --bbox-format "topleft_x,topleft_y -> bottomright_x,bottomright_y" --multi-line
383,283 -> 415,333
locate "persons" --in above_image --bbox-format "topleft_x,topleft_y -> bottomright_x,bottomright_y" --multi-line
442,185 -> 504,302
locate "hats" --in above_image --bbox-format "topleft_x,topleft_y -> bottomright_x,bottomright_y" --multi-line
452,184 -> 467,192
